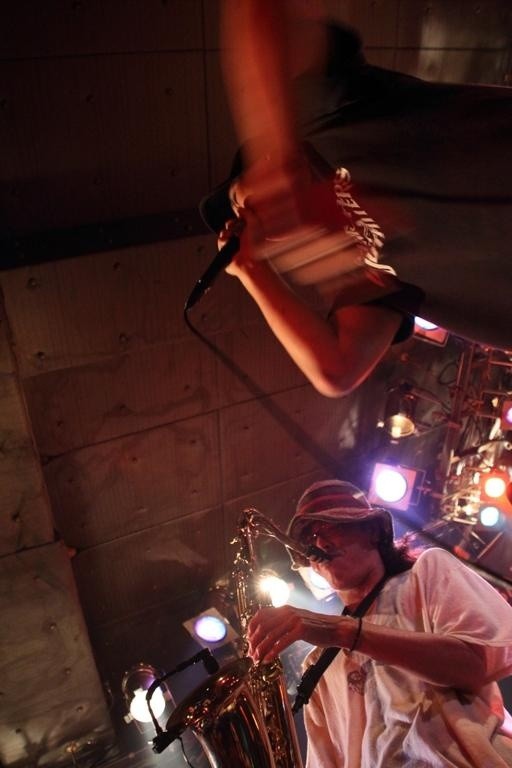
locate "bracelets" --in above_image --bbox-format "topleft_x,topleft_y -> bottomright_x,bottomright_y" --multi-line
349,618 -> 363,653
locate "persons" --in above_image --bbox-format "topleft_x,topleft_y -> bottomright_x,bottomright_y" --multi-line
196,1 -> 512,401
245,478 -> 512,767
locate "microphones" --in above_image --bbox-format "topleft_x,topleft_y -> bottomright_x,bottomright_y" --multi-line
200,651 -> 219,675
186,210 -> 248,313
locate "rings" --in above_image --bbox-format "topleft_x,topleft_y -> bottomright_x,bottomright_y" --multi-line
273,639 -> 284,651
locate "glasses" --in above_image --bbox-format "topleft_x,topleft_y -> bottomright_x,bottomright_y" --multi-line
297,521 -> 363,547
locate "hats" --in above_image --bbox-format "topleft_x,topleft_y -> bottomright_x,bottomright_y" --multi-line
286,480 -> 394,571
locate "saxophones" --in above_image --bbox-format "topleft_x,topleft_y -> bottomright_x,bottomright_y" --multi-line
167,510 -> 329,767
